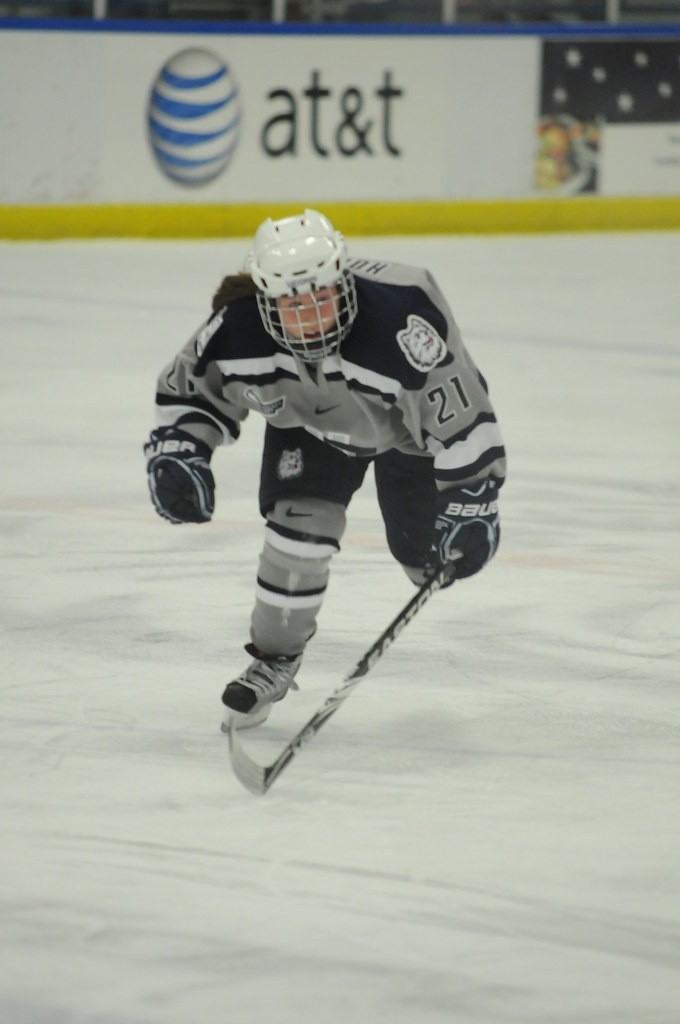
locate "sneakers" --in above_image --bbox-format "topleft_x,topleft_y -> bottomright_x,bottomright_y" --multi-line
221,643 -> 302,732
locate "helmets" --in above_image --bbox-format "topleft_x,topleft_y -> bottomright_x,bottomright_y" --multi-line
251,208 -> 346,297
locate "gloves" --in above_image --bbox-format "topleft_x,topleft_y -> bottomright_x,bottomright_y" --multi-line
143,426 -> 215,524
432,478 -> 500,580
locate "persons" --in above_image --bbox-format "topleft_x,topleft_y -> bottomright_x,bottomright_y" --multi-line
145,209 -> 507,730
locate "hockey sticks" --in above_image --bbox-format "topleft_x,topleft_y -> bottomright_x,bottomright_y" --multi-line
228,561 -> 455,797
